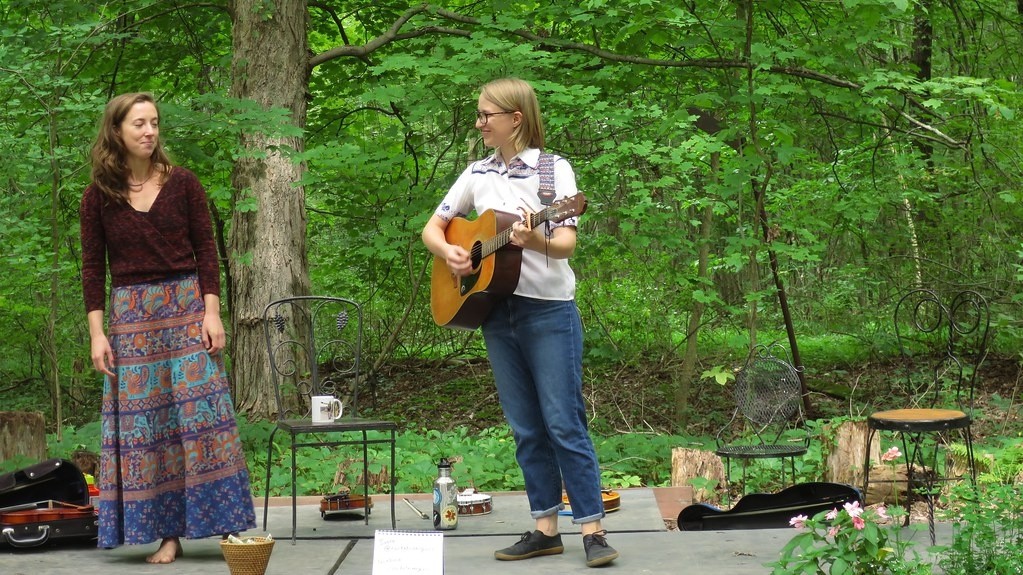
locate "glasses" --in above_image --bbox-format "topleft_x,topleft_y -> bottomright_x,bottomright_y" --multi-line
475,111 -> 514,124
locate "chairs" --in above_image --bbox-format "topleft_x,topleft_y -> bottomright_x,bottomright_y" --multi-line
861,289 -> 990,547
716,342 -> 810,510
262,294 -> 399,545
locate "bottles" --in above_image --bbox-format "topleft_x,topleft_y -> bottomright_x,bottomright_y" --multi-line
84,473 -> 100,513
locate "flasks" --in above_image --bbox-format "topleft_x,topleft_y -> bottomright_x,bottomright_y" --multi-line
432,458 -> 459,529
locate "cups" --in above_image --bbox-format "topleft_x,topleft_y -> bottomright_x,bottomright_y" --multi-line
311,396 -> 343,424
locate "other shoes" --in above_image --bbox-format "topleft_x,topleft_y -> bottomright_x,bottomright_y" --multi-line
583,529 -> 618,566
495,530 -> 564,560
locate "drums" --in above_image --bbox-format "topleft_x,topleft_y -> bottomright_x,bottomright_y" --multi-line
458,493 -> 494,517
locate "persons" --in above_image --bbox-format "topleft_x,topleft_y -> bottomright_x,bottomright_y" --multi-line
421,78 -> 620,567
78,92 -> 256,564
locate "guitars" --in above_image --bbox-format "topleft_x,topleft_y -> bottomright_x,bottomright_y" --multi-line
557,485 -> 621,516
430,191 -> 588,331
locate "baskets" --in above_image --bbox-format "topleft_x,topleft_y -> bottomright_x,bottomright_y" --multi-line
220,536 -> 275,575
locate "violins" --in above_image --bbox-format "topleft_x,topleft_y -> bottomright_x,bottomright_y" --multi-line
320,486 -> 373,521
0,498 -> 94,523
